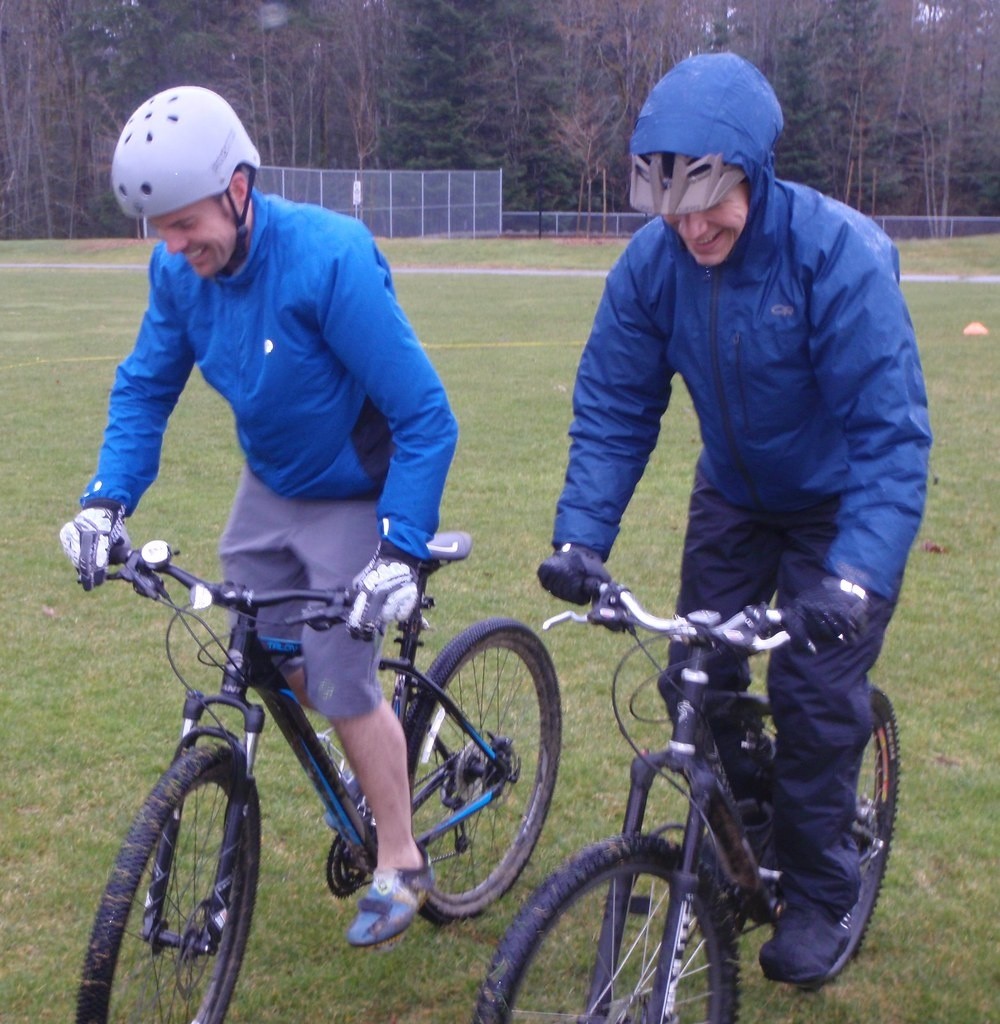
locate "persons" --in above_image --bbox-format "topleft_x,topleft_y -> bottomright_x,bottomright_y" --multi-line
59,81 -> 467,947
531,49 -> 936,986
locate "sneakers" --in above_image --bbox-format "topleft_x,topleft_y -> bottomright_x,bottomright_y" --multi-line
344,847 -> 440,947
757,902 -> 848,981
320,766 -> 366,833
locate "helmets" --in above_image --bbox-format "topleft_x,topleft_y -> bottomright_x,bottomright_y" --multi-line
625,143 -> 751,214
110,88 -> 259,218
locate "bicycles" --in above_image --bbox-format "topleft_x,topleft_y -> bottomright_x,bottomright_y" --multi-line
75,531 -> 565,1023
471,575 -> 899,1024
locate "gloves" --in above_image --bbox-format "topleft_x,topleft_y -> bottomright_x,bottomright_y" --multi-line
61,493 -> 129,589
341,551 -> 428,643
539,546 -> 609,603
786,569 -> 871,650
